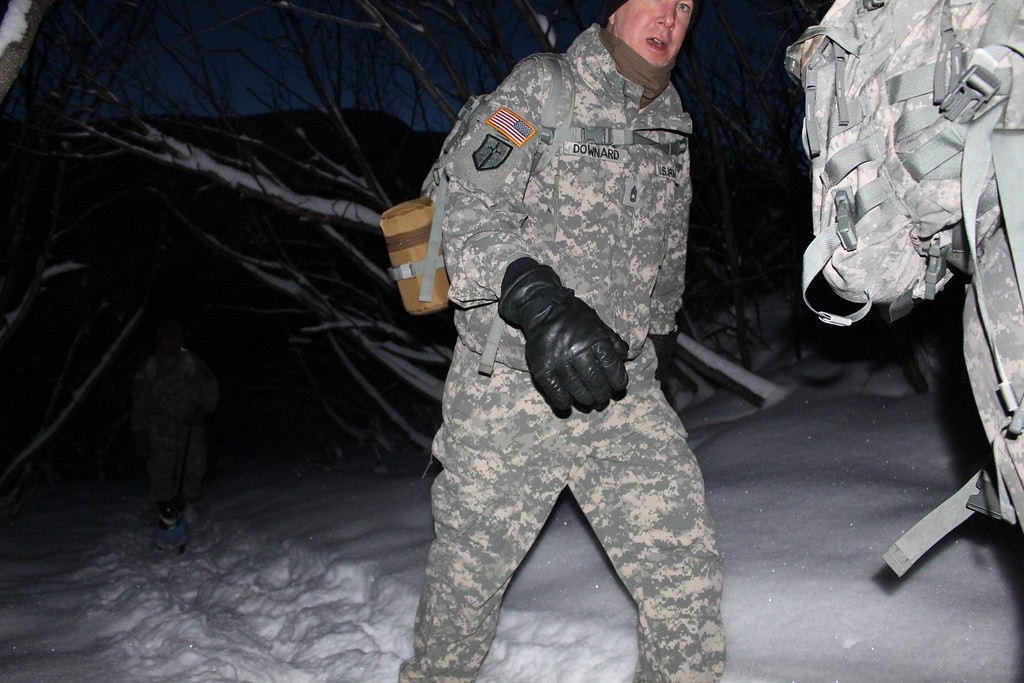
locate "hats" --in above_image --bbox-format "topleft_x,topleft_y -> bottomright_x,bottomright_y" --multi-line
603,0 -> 629,26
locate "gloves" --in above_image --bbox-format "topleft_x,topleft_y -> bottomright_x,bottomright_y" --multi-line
498,256 -> 630,409
647,323 -> 682,392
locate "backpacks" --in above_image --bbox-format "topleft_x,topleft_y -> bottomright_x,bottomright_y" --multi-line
800,0 -> 1024,322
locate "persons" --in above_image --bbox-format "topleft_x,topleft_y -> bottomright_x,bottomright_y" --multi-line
963,0 -> 1024,534
400,0 -> 726,683
